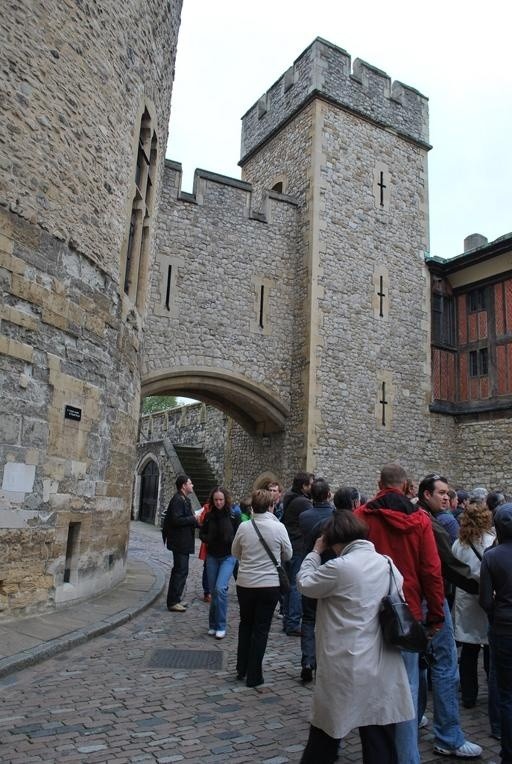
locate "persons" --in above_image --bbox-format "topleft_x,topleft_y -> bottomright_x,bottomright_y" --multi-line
160,474 -> 202,612
197,463 -> 511,763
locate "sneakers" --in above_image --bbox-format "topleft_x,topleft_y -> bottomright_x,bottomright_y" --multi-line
418,715 -> 427,728
169,595 -> 312,688
433,741 -> 482,757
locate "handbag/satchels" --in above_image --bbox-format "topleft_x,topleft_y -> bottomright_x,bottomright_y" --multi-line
276,564 -> 290,593
380,593 -> 426,649
